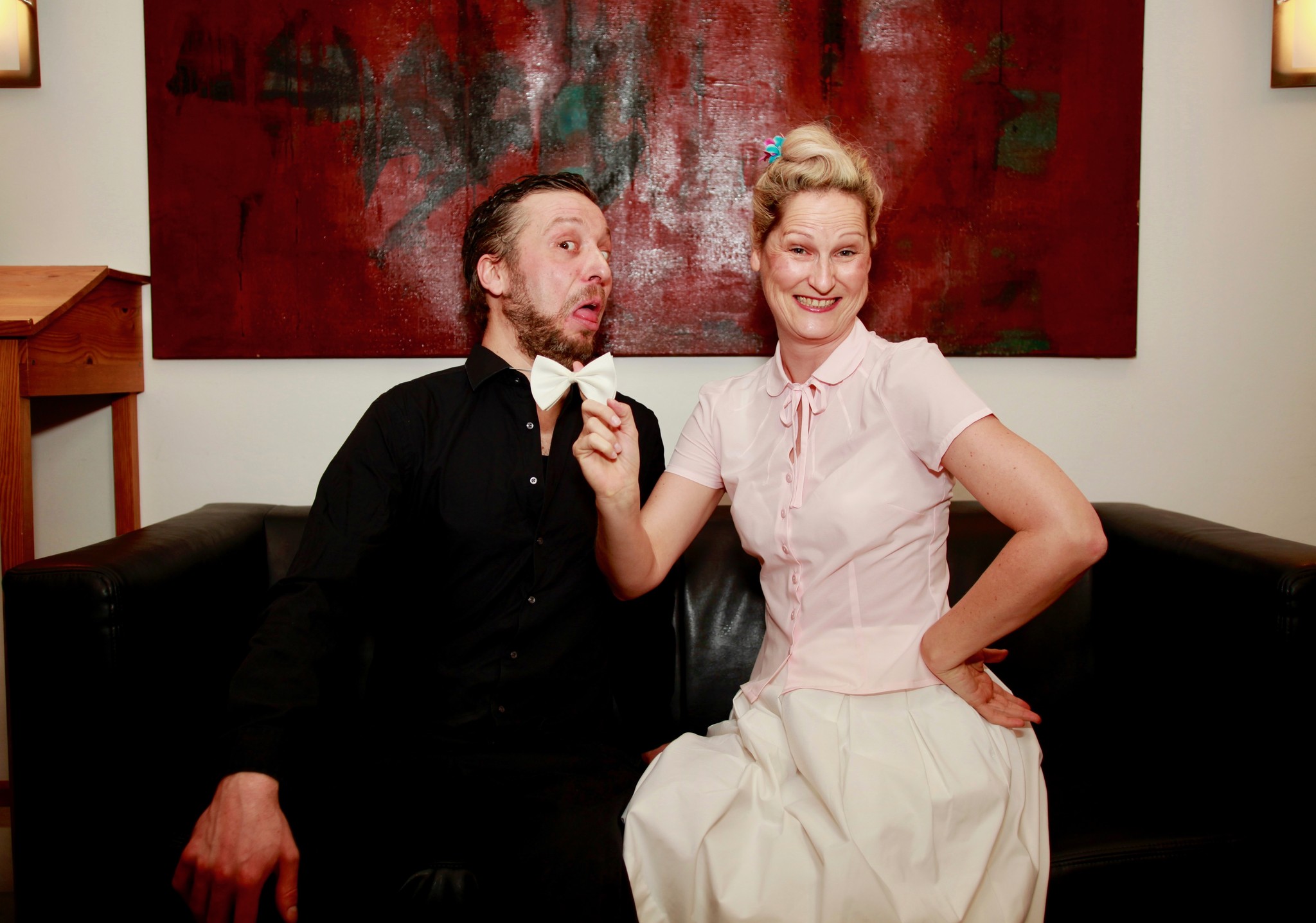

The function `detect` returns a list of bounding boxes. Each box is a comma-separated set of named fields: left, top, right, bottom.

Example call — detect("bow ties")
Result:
left=531, top=349, right=621, bottom=414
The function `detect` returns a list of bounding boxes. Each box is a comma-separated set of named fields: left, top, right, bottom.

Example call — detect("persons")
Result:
left=164, top=170, right=681, bottom=923
left=574, top=124, right=1108, bottom=923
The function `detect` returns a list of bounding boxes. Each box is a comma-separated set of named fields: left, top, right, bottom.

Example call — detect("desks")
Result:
left=0, top=264, right=155, bottom=571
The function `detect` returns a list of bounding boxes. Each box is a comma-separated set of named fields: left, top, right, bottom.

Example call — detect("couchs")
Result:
left=1, top=497, right=1316, bottom=923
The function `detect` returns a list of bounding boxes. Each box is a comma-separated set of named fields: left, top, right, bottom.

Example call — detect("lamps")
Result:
left=0, top=0, right=42, bottom=91
left=1265, top=0, right=1316, bottom=89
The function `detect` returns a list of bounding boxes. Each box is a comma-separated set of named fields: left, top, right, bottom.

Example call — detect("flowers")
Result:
left=758, top=132, right=788, bottom=166
